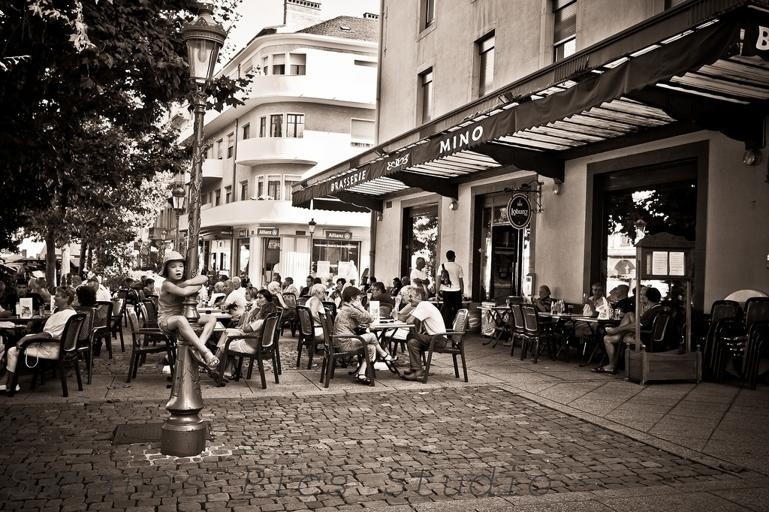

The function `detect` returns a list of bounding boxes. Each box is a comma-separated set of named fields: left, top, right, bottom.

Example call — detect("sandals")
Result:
left=188, top=346, right=206, bottom=366
left=203, top=351, right=220, bottom=367
left=353, top=373, right=370, bottom=384
left=379, top=353, right=398, bottom=362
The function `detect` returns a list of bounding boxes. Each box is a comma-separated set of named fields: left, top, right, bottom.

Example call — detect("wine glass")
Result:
left=582, top=292, right=589, bottom=300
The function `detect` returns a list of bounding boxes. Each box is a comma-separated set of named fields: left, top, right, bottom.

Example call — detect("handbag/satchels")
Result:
left=441, top=270, right=449, bottom=284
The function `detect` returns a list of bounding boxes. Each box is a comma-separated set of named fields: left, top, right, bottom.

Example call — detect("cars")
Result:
left=0, top=253, right=87, bottom=300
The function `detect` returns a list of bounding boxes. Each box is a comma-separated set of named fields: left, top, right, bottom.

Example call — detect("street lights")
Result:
left=154, top=7, right=232, bottom=458
left=170, top=184, right=186, bottom=249
left=306, top=216, right=317, bottom=275
left=161, top=230, right=167, bottom=243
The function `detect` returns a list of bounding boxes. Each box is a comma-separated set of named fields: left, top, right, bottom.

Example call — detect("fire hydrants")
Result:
left=137, top=237, right=143, bottom=268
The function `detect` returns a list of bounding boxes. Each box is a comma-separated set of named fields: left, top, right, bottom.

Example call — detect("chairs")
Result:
left=0, top=284, right=768, bottom=399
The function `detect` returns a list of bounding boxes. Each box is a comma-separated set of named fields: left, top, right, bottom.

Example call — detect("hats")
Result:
left=159, top=251, right=185, bottom=277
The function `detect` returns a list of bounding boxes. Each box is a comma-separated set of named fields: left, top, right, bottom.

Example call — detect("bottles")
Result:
left=551, top=298, right=565, bottom=315
left=38, top=305, right=45, bottom=316
left=200, top=298, right=229, bottom=315
left=15, top=301, right=23, bottom=316
left=610, top=300, right=621, bottom=321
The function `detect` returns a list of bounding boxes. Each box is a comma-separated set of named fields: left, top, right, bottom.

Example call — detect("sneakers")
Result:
left=404, top=368, right=425, bottom=381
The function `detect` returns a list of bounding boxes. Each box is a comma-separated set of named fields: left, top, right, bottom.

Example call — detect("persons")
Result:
left=434, top=251, right=463, bottom=340
left=556, top=283, right=664, bottom=374
left=504, top=285, right=552, bottom=346
left=134, top=250, right=277, bottom=381
left=268, top=257, right=448, bottom=385
left=1, top=258, right=132, bottom=390
